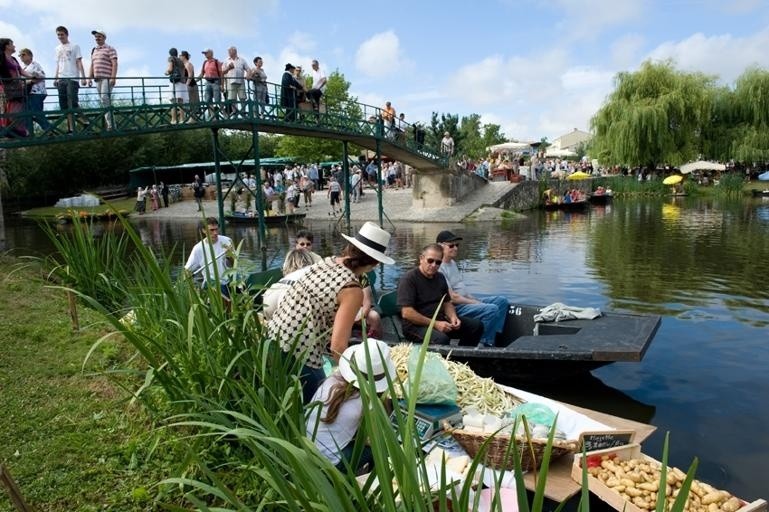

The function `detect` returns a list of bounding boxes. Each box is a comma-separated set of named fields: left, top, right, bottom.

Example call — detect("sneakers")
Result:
left=185, top=116, right=198, bottom=125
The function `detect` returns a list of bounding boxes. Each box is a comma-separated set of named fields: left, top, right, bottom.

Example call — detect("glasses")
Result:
left=300, top=243, right=310, bottom=246
left=443, top=242, right=459, bottom=248
left=18, top=54, right=25, bottom=57
left=428, top=259, right=441, bottom=265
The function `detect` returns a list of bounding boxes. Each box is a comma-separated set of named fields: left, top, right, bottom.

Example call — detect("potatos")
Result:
left=587, top=454, right=742, bottom=512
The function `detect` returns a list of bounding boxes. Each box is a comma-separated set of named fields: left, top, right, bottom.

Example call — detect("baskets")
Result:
left=442, top=414, right=581, bottom=471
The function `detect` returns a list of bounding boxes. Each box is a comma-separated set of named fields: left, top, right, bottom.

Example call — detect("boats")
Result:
left=57, top=210, right=129, bottom=224
left=193, top=286, right=661, bottom=378
left=225, top=214, right=305, bottom=224
left=752, top=189, right=769, bottom=196
left=542, top=192, right=615, bottom=213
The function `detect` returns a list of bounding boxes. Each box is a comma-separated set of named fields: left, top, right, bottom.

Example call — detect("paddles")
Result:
left=119, top=246, right=232, bottom=327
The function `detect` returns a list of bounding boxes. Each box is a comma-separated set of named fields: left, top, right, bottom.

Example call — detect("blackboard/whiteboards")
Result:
left=577, top=431, right=636, bottom=452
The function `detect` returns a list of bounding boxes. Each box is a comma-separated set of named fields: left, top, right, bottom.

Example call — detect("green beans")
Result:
left=391, top=341, right=520, bottom=416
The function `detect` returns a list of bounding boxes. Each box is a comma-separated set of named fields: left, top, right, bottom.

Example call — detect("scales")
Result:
left=387, top=399, right=462, bottom=441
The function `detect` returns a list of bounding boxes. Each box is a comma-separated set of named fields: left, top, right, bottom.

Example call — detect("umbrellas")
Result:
left=565, top=171, right=591, bottom=182
left=663, top=175, right=683, bottom=190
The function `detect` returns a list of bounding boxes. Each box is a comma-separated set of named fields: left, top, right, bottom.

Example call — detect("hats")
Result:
left=91, top=30, right=107, bottom=39
left=285, top=64, right=295, bottom=71
left=339, top=337, right=396, bottom=393
left=201, top=48, right=213, bottom=53
left=437, top=231, right=463, bottom=242
left=341, top=221, right=396, bottom=265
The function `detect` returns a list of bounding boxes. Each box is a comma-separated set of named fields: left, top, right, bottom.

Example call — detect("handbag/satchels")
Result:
left=170, top=57, right=181, bottom=83
left=4, top=80, right=24, bottom=101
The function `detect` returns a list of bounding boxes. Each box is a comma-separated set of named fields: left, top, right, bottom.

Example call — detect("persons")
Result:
left=180, top=217, right=235, bottom=300
left=0, top=25, right=118, bottom=138
left=165, top=47, right=326, bottom=124
left=460, top=153, right=565, bottom=182
left=543, top=187, right=613, bottom=205
left=368, top=102, right=455, bottom=159
left=565, top=159, right=593, bottom=175
left=262, top=221, right=511, bottom=476
left=135, top=154, right=412, bottom=214
